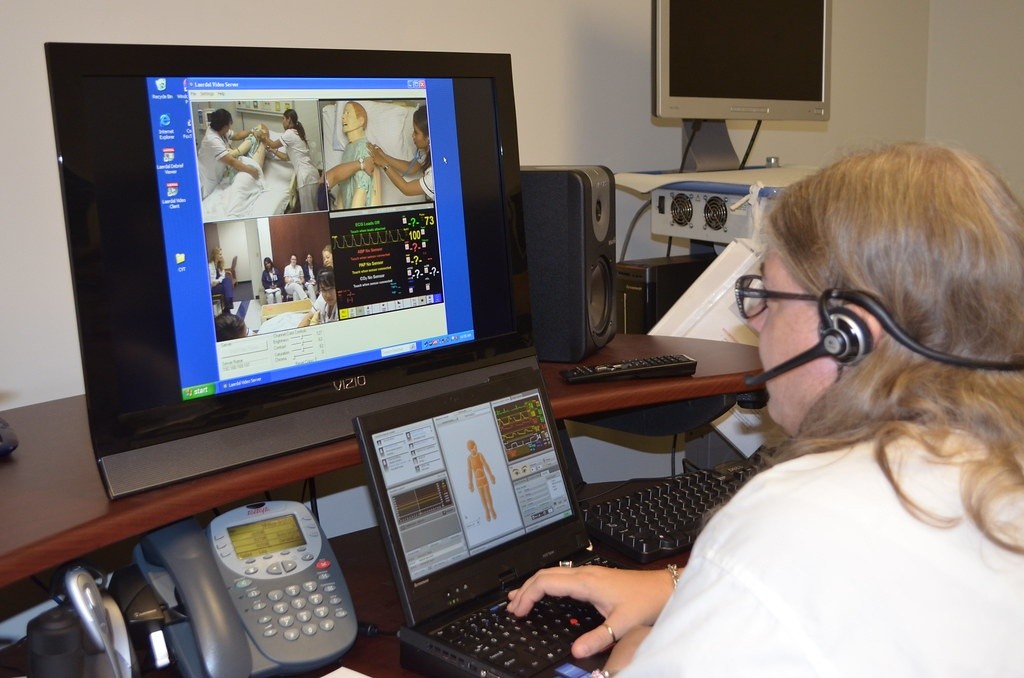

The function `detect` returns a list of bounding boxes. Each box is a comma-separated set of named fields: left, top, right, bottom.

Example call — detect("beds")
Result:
left=202, top=127, right=316, bottom=223
left=322, top=103, right=427, bottom=209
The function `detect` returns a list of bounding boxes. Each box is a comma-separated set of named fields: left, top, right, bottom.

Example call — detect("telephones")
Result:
left=135, top=498, right=363, bottom=677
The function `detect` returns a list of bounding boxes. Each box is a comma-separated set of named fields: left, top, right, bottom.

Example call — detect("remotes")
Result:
left=558, top=354, right=698, bottom=383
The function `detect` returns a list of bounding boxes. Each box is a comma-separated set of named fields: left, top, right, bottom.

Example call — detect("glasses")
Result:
left=735, top=275, right=820, bottom=319
left=318, top=286, right=336, bottom=293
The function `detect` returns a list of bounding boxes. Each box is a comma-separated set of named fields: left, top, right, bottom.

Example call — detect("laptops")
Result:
left=351, top=368, right=626, bottom=678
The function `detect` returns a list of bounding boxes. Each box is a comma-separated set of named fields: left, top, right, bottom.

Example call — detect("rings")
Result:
left=604, top=623, right=617, bottom=642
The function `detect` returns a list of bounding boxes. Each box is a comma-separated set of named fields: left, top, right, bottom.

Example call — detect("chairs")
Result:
left=224, top=255, right=238, bottom=288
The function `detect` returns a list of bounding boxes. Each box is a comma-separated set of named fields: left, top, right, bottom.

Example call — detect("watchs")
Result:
left=359, top=157, right=364, bottom=170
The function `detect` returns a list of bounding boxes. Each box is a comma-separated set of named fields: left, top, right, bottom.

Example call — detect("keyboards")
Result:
left=580, top=457, right=761, bottom=563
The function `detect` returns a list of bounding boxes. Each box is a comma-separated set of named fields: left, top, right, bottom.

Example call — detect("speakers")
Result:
left=523, top=164, right=618, bottom=366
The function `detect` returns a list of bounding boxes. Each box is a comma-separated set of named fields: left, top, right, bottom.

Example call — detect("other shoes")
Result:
left=226, top=301, right=233, bottom=310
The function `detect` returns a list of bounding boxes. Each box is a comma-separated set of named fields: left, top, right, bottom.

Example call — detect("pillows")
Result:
left=332, top=101, right=420, bottom=161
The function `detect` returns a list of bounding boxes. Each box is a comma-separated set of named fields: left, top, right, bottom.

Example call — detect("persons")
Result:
left=366, top=105, right=436, bottom=202
left=196, top=108, right=260, bottom=199
left=325, top=156, right=376, bottom=190
left=215, top=310, right=251, bottom=343
left=262, top=245, right=340, bottom=326
left=229, top=120, right=269, bottom=212
left=334, top=101, right=376, bottom=208
left=207, top=247, right=235, bottom=313
left=253, top=109, right=321, bottom=213
left=507, top=139, right=1020, bottom=678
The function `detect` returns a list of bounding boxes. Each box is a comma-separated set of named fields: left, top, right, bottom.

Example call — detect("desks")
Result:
left=0, top=336, right=765, bottom=678
left=261, top=298, right=314, bottom=319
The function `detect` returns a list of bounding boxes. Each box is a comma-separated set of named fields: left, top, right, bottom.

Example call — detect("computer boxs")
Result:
left=616, top=253, right=718, bottom=334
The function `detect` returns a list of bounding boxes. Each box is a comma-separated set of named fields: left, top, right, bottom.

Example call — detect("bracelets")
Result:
left=384, top=165, right=390, bottom=171
left=667, top=563, right=680, bottom=589
left=591, top=669, right=610, bottom=678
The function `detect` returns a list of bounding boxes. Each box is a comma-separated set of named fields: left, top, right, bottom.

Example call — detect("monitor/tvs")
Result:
left=44, top=42, right=544, bottom=499
left=650, top=0, right=833, bottom=171
